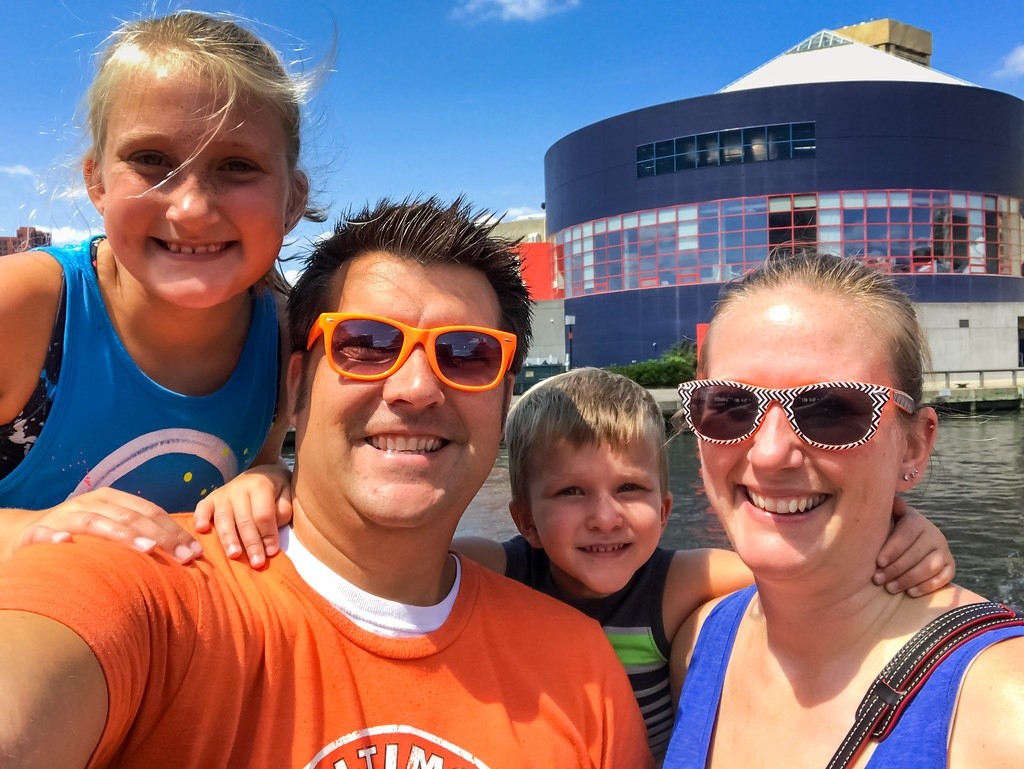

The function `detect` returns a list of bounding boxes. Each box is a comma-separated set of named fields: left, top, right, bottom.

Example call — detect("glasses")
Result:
left=677, top=379, right=915, bottom=452
left=307, top=312, right=517, bottom=392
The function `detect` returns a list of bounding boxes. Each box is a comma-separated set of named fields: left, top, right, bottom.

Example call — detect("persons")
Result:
left=661, top=246, right=1023, bottom=769
left=0, top=191, right=650, bottom=769
left=192, top=366, right=958, bottom=769
left=0, top=0, right=347, bottom=565
